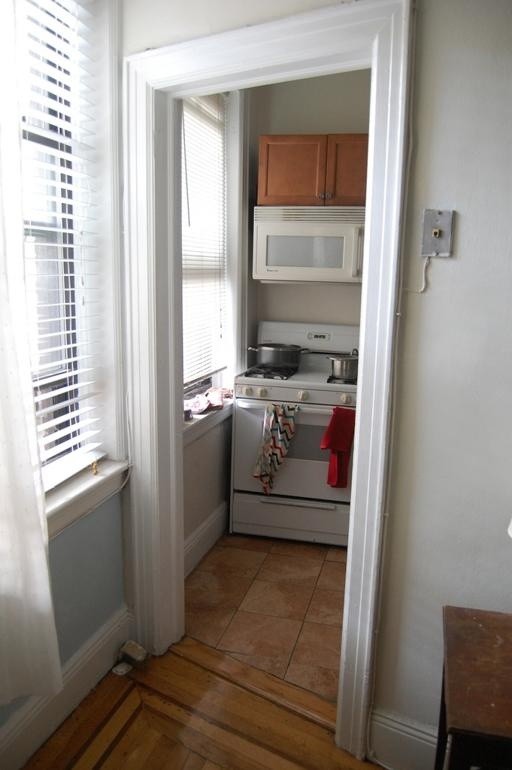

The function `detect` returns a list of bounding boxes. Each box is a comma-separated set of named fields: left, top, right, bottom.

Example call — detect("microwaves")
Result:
left=249, top=207, right=367, bottom=288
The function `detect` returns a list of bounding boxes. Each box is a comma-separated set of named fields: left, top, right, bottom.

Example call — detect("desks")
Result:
left=436, top=604, right=511, bottom=768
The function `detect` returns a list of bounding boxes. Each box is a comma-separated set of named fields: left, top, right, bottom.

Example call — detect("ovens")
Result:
left=229, top=399, right=359, bottom=550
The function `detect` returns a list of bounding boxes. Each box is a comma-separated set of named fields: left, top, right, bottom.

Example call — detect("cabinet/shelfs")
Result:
left=256, top=132, right=370, bottom=204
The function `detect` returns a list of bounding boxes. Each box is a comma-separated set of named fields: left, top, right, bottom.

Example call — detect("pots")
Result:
left=247, top=343, right=312, bottom=369
left=326, top=348, right=358, bottom=379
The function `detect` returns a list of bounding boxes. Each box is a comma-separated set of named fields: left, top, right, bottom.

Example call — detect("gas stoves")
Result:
left=233, top=367, right=357, bottom=407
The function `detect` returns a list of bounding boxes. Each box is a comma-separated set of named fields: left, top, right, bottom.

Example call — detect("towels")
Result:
left=250, top=404, right=355, bottom=497
